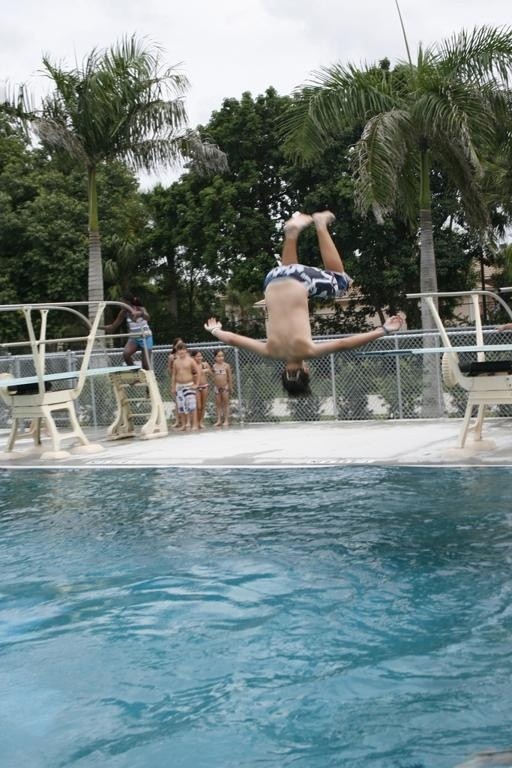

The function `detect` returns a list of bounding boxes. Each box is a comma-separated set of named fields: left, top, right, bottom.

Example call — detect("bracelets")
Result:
left=381, top=326, right=389, bottom=334
left=140, top=311, right=144, bottom=317
left=211, top=327, right=219, bottom=335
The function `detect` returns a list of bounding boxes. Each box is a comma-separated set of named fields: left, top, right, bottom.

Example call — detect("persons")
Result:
left=203, top=210, right=404, bottom=397
left=166, top=337, right=232, bottom=432
left=496, top=323, right=512, bottom=332
left=98, top=295, right=153, bottom=398
